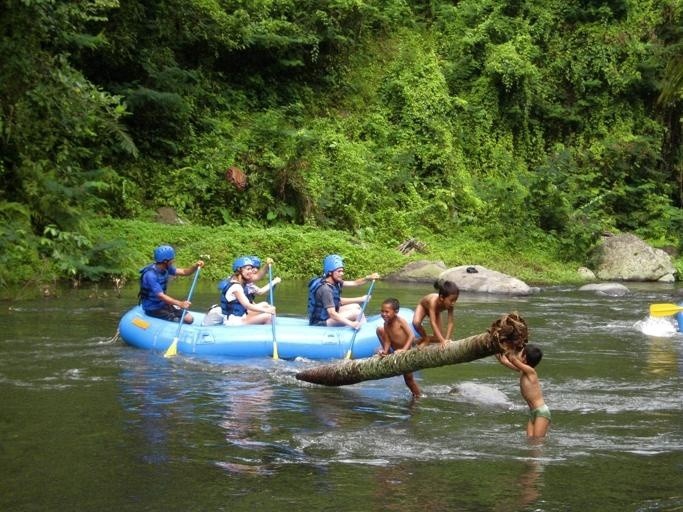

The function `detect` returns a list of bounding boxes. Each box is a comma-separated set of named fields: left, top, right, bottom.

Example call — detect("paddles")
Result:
left=166, top=265, right=200, bottom=355
left=269, top=263, right=278, bottom=360
left=650, top=304, right=683, bottom=316
left=345, top=279, right=376, bottom=360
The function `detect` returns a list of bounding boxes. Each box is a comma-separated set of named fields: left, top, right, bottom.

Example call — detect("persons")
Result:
left=320, top=254, right=381, bottom=306
left=306, top=259, right=372, bottom=332
left=241, top=254, right=282, bottom=305
left=216, top=255, right=277, bottom=328
left=410, top=278, right=461, bottom=352
left=374, top=297, right=421, bottom=399
left=495, top=342, right=552, bottom=438
left=136, top=243, right=204, bottom=326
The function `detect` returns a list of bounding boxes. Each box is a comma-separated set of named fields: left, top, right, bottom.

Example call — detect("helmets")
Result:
left=154, top=246, right=175, bottom=263
left=324, top=258, right=344, bottom=272
left=249, top=256, right=261, bottom=269
left=323, top=254, right=342, bottom=265
left=232, top=257, right=253, bottom=272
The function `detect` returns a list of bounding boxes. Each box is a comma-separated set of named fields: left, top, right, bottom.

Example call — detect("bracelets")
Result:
left=364, top=274, right=370, bottom=281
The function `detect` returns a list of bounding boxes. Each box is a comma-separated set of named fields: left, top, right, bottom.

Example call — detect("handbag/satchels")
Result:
left=200, top=304, right=223, bottom=325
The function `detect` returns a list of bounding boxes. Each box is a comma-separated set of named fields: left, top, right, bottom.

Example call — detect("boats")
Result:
left=119, top=304, right=421, bottom=363
